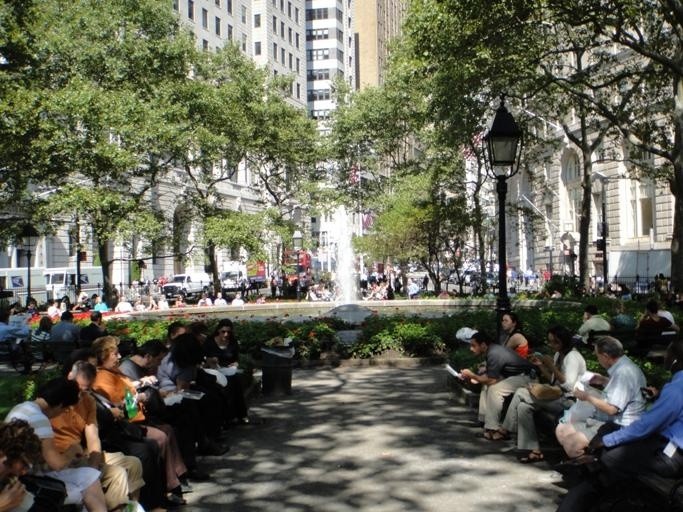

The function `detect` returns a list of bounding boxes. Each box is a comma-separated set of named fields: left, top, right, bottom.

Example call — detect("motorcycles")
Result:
left=511, top=270, right=537, bottom=288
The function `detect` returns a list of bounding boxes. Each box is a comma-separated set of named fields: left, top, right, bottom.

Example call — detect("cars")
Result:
left=430, top=266, right=497, bottom=296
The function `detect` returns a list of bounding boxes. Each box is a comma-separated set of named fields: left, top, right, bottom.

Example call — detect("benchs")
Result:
left=577, top=329, right=676, bottom=365
left=542, top=370, right=683, bottom=511
left=0, top=340, right=137, bottom=373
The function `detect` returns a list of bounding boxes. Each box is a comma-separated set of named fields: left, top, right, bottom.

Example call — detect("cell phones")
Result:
left=530, top=367, right=538, bottom=378
left=534, top=351, right=543, bottom=358
left=642, top=389, right=654, bottom=398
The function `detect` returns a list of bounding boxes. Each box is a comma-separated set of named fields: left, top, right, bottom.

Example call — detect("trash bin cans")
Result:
left=258, top=343, right=294, bottom=397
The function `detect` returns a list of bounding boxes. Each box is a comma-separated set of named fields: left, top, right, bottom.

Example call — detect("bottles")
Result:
left=124, top=387, right=138, bottom=420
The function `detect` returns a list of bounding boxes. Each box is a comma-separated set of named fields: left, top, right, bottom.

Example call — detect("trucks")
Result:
left=0, top=267, right=102, bottom=310
left=218, top=265, right=264, bottom=290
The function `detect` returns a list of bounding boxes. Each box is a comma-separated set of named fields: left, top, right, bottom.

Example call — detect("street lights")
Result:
left=292, top=230, right=302, bottom=302
left=484, top=95, right=523, bottom=343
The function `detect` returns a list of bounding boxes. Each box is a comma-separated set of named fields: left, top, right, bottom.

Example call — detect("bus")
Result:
left=280, top=249, right=311, bottom=290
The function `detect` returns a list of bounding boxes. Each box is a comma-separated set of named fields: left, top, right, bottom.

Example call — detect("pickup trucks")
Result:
left=165, top=274, right=213, bottom=298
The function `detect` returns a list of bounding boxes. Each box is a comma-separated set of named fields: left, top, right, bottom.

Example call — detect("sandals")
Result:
left=520, top=450, right=546, bottom=464
left=483, top=428, right=512, bottom=442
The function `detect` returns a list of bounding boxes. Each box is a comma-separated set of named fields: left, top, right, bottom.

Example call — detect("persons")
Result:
left=510, top=267, right=549, bottom=288
left=198, top=291, right=265, bottom=306
left=271, top=270, right=335, bottom=301
left=361, top=275, right=461, bottom=300
left=537, top=284, right=631, bottom=300
left=2, top=318, right=251, bottom=511
left=462, top=302, right=683, bottom=512
left=131, top=278, right=186, bottom=311
left=1, top=289, right=131, bottom=370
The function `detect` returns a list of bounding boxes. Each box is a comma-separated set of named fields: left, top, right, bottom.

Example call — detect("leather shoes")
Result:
left=554, top=456, right=596, bottom=473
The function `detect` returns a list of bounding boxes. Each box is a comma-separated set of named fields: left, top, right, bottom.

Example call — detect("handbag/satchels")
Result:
left=117, top=419, right=143, bottom=441
left=20, top=472, right=67, bottom=512
left=136, top=383, right=170, bottom=426
left=530, top=382, right=564, bottom=402
